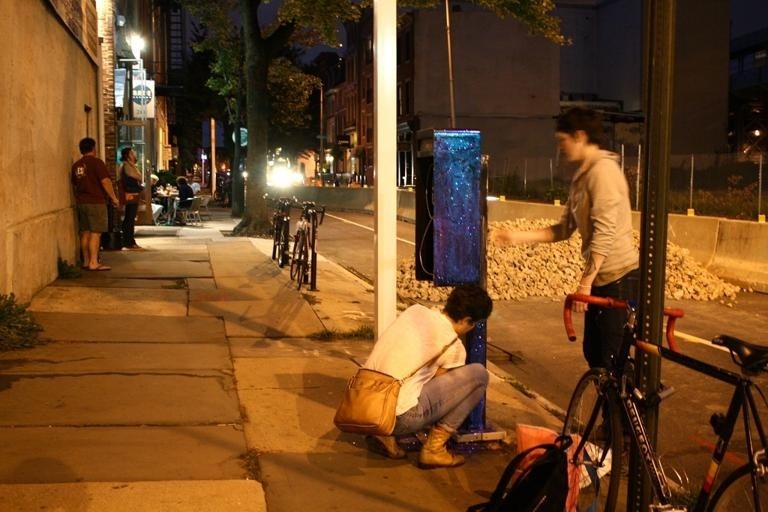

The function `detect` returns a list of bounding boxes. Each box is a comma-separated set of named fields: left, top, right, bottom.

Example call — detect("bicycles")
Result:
left=261, top=192, right=326, bottom=291
left=498, top=293, right=768, bottom=511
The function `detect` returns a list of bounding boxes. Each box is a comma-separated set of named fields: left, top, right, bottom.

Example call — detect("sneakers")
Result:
left=127, top=242, right=142, bottom=248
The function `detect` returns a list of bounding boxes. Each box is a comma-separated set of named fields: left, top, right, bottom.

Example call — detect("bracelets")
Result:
left=578, top=282, right=592, bottom=289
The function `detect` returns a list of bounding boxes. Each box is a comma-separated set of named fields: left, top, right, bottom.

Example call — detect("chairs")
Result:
left=200, top=195, right=211, bottom=208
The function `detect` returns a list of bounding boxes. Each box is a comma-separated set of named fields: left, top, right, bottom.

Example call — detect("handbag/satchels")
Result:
left=333, top=368, right=403, bottom=436
left=120, top=164, right=144, bottom=193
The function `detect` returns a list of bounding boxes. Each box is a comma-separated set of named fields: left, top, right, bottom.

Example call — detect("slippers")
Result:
left=81, top=263, right=111, bottom=270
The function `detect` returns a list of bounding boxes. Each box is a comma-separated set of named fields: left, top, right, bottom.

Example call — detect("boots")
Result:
left=418, top=423, right=465, bottom=469
left=366, top=435, right=407, bottom=459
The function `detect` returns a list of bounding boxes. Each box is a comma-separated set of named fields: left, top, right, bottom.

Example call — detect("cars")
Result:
left=204, top=170, right=231, bottom=189
left=239, top=160, right=247, bottom=184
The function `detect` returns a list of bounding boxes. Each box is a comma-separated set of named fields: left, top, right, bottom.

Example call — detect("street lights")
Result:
left=288, top=75, right=324, bottom=173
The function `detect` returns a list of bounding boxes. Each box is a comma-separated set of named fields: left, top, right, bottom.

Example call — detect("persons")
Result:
left=492, top=112, right=639, bottom=445
left=72, top=138, right=120, bottom=271
left=365, top=285, right=493, bottom=469
left=151, top=174, right=193, bottom=225
left=120, top=147, right=143, bottom=250
